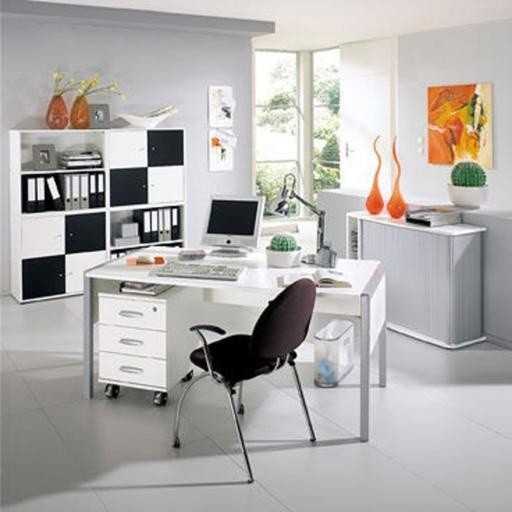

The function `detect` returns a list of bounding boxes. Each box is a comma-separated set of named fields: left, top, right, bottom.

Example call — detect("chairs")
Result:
left=175, top=279, right=316, bottom=482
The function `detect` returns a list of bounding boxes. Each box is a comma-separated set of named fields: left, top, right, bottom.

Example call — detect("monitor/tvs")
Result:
left=201, top=195, right=267, bottom=257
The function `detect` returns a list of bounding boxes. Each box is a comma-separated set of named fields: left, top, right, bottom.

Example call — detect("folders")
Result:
left=22, top=172, right=105, bottom=213
left=133, top=207, right=180, bottom=243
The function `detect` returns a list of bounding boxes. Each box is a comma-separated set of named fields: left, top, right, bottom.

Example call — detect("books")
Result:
left=59, top=148, right=103, bottom=170
left=118, top=284, right=174, bottom=296
left=276, top=269, right=352, bottom=287
left=123, top=280, right=155, bottom=289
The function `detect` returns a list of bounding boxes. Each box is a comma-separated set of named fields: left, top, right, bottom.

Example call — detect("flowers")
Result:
left=53, top=71, right=128, bottom=101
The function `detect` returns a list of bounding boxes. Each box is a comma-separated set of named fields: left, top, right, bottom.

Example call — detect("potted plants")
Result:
left=266, top=233, right=302, bottom=268
left=447, top=161, right=489, bottom=208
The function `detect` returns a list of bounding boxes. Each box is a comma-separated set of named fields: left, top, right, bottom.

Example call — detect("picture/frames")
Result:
left=87, top=104, right=111, bottom=129
left=207, top=85, right=236, bottom=172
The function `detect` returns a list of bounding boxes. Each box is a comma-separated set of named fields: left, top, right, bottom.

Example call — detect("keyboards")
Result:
left=154, top=261, right=248, bottom=282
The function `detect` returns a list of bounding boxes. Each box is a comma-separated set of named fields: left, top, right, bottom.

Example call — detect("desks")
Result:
left=84, top=245, right=387, bottom=443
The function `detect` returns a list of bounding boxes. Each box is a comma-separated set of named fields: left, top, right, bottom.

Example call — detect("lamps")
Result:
left=265, top=173, right=337, bottom=268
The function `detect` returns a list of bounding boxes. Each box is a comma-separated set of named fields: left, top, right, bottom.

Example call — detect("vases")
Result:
left=46, top=96, right=90, bottom=129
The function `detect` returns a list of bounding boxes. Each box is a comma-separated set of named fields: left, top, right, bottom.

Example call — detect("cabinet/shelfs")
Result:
left=8, top=129, right=185, bottom=306
left=96, top=289, right=195, bottom=394
left=345, top=209, right=487, bottom=351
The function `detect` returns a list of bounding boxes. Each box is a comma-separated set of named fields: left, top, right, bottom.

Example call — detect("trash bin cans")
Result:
left=314, top=319, right=355, bottom=388
